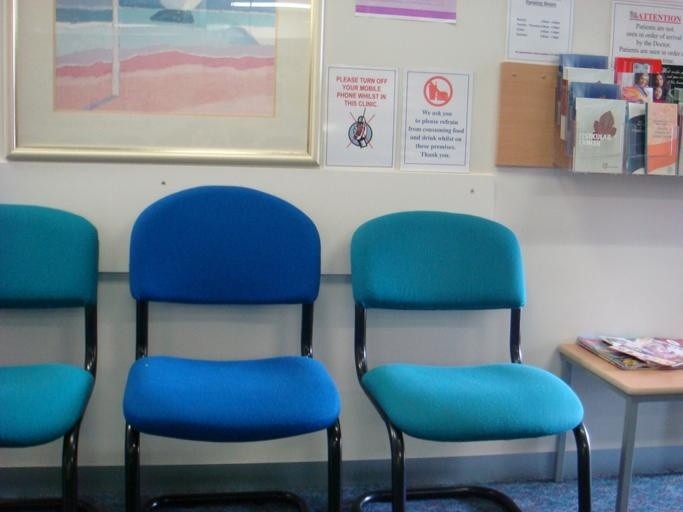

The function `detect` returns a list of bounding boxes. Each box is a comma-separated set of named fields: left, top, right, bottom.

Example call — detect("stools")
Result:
left=555, top=341, right=683, bottom=511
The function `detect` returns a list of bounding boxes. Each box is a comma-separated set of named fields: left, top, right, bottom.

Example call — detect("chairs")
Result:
left=2, top=204, right=100, bottom=508
left=349, top=210, right=591, bottom=512
left=122, top=183, right=344, bottom=511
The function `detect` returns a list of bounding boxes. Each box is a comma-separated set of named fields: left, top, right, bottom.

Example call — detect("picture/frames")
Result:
left=0, top=0, right=325, bottom=168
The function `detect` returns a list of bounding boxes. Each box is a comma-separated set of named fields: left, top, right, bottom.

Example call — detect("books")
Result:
left=574, top=333, right=683, bottom=372
left=551, top=51, right=682, bottom=177
